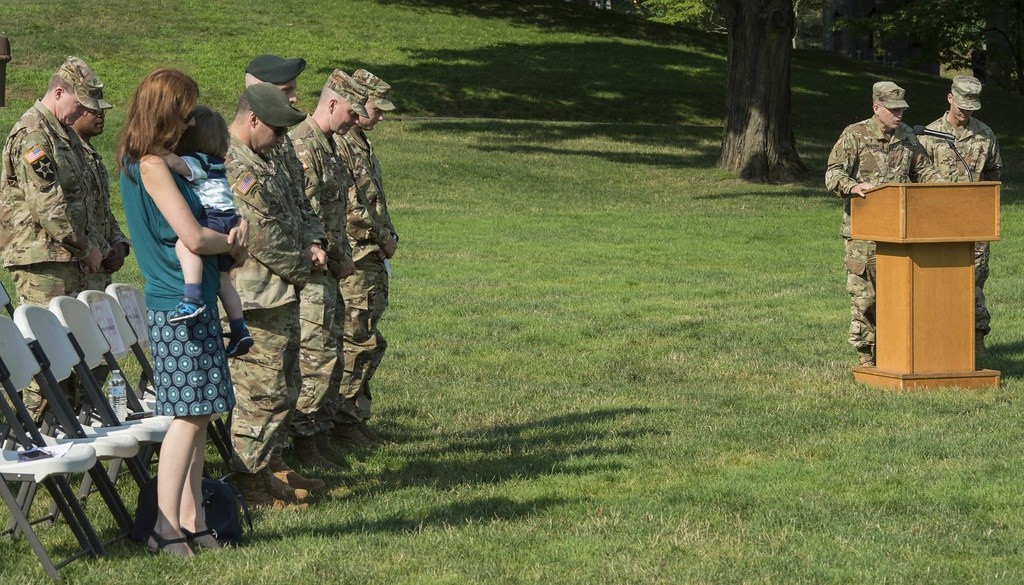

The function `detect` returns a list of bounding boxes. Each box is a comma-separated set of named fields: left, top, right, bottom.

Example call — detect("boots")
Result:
left=241, top=480, right=308, bottom=512
left=316, top=432, right=346, bottom=465
left=265, top=475, right=312, bottom=503
left=857, top=347, right=875, bottom=367
left=335, top=421, right=384, bottom=450
left=294, top=437, right=341, bottom=471
left=270, top=457, right=322, bottom=489
left=975, top=330, right=988, bottom=357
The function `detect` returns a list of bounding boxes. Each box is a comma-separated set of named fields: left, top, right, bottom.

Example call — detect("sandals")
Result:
left=145, top=530, right=187, bottom=558
left=183, top=528, right=213, bottom=553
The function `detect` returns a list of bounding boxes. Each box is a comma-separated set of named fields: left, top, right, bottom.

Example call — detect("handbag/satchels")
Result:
left=131, top=474, right=242, bottom=547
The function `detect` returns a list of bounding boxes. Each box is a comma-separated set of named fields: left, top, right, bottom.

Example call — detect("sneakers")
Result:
left=225, top=329, right=254, bottom=357
left=169, top=297, right=206, bottom=322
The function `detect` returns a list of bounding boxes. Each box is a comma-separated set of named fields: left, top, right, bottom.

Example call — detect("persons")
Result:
left=119, top=70, right=249, bottom=560
left=285, top=67, right=368, bottom=464
left=826, top=79, right=947, bottom=368
left=215, top=52, right=330, bottom=511
left=0, top=56, right=133, bottom=447
left=153, top=104, right=256, bottom=360
left=908, top=71, right=1004, bottom=364
left=322, top=68, right=401, bottom=448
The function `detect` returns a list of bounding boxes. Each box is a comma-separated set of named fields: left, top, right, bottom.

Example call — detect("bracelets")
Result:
left=390, top=231, right=400, bottom=241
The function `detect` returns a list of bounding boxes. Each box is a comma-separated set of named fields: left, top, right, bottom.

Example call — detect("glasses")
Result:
left=182, top=108, right=197, bottom=124
left=273, top=126, right=289, bottom=136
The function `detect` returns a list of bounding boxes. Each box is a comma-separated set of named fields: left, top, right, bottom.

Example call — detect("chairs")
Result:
left=0, top=280, right=233, bottom=585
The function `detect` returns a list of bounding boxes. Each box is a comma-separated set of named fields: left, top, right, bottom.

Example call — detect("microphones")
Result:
left=913, top=125, right=956, bottom=140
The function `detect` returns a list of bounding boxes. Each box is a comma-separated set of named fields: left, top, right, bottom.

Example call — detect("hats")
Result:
left=873, top=81, right=909, bottom=109
left=324, top=69, right=369, bottom=118
left=247, top=82, right=307, bottom=127
left=59, top=55, right=104, bottom=110
left=951, top=75, right=982, bottom=111
left=352, top=69, right=396, bottom=111
left=245, top=54, right=306, bottom=84
left=97, top=95, right=113, bottom=109
